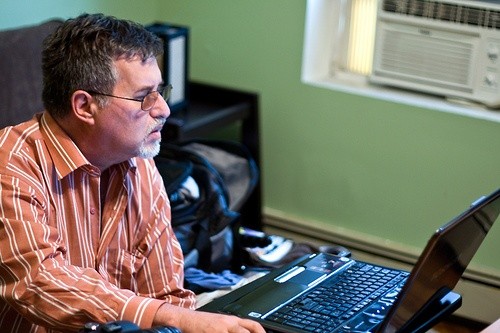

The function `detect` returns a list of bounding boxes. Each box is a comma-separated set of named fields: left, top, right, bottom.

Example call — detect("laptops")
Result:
left=222, top=189, right=497, bottom=333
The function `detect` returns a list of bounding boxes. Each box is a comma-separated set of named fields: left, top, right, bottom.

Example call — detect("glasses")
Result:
left=88, top=83, right=173, bottom=111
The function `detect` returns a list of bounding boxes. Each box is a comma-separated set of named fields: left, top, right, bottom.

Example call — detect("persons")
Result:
left=0, top=12, right=268, bottom=333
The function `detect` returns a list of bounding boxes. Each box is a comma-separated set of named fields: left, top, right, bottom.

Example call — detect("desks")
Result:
left=162, top=81, right=262, bottom=233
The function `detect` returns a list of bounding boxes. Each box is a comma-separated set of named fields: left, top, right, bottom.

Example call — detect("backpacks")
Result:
left=153, top=138, right=259, bottom=296
